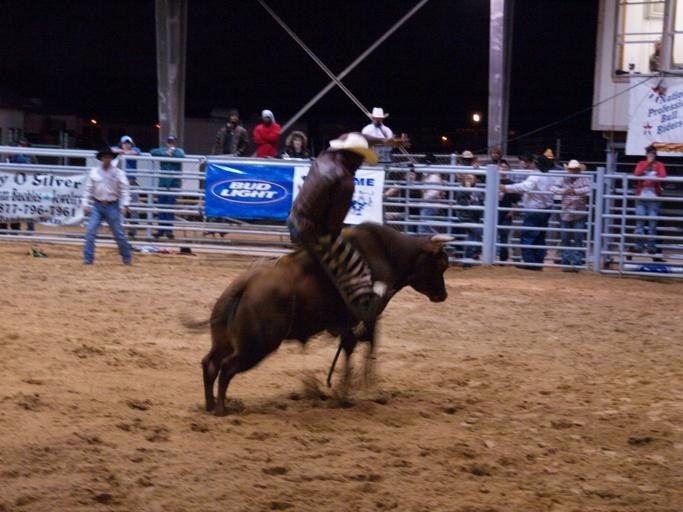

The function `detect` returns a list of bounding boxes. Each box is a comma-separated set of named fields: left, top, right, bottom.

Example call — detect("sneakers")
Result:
left=124, top=231, right=176, bottom=239
left=463, top=247, right=661, bottom=269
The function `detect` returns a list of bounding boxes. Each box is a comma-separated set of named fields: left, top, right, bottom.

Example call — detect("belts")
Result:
left=91, top=196, right=119, bottom=205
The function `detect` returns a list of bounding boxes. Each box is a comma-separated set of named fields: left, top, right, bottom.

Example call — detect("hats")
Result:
left=284, top=130, right=309, bottom=149
left=96, top=146, right=120, bottom=161
left=167, top=135, right=178, bottom=142
left=563, top=159, right=587, bottom=172
left=329, top=133, right=379, bottom=167
left=543, top=148, right=554, bottom=160
left=461, top=150, right=474, bottom=159
left=120, top=136, right=134, bottom=144
left=367, top=106, right=390, bottom=120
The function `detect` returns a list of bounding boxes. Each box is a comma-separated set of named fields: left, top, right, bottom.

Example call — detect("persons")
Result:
left=0, top=140, right=24, bottom=234
left=106, top=136, right=142, bottom=239
left=147, top=136, right=183, bottom=238
left=16, top=138, right=37, bottom=232
left=250, top=110, right=279, bottom=157
left=282, top=132, right=410, bottom=341
left=210, top=110, right=249, bottom=157
left=361, top=107, right=394, bottom=163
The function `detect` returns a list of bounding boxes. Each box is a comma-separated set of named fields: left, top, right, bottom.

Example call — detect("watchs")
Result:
left=81, top=144, right=130, bottom=265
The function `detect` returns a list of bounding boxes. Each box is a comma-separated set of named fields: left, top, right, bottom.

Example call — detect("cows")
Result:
left=177, top=220, right=455, bottom=418
